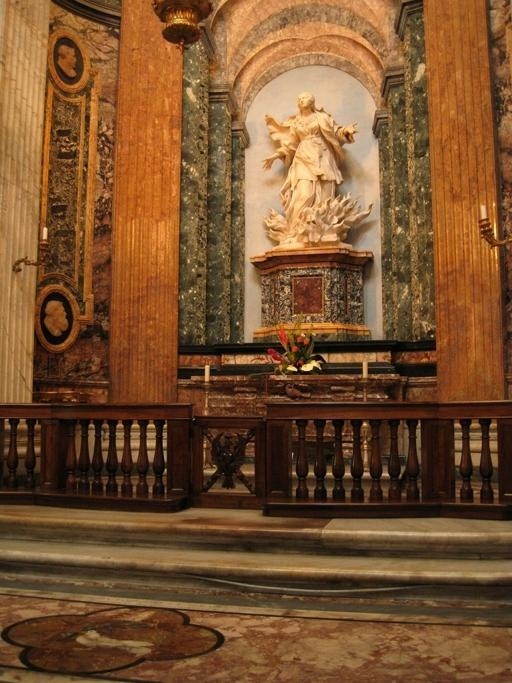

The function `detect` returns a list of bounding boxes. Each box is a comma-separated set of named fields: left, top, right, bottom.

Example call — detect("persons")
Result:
left=261, top=91, right=360, bottom=212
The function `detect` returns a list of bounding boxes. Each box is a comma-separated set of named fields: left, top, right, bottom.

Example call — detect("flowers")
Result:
left=250, top=310, right=326, bottom=375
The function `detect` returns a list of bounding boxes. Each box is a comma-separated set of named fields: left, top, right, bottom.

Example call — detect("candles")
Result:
left=42, top=225, right=49, bottom=240
left=362, top=359, right=369, bottom=378
left=204, top=364, right=210, bottom=383
left=479, top=204, right=487, bottom=220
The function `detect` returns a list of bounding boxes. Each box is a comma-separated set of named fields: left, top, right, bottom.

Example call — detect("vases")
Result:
left=285, top=383, right=313, bottom=400
